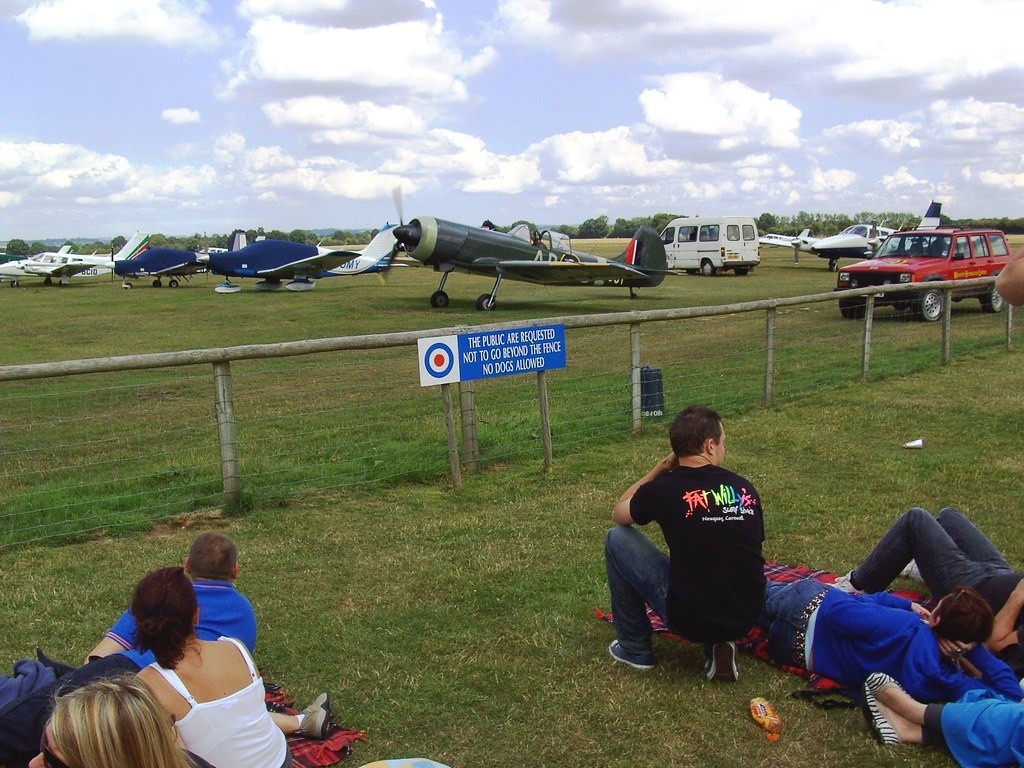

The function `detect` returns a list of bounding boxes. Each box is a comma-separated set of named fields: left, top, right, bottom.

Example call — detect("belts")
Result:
left=793, top=590, right=829, bottom=670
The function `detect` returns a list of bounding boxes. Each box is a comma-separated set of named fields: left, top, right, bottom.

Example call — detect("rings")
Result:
left=955, top=649, right=962, bottom=654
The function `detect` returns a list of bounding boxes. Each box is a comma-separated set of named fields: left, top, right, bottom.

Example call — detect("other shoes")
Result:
left=861, top=683, right=899, bottom=746
left=866, top=672, right=923, bottom=708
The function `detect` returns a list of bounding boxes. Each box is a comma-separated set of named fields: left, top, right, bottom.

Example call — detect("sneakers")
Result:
left=705, top=639, right=740, bottom=683
left=824, top=569, right=864, bottom=595
left=294, top=693, right=331, bottom=739
left=902, top=560, right=923, bottom=582
left=608, top=637, right=657, bottom=669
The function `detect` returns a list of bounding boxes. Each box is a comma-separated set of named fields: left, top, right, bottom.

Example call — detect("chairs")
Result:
left=709, top=229, right=715, bottom=236
left=910, top=243, right=923, bottom=254
left=701, top=231, right=707, bottom=235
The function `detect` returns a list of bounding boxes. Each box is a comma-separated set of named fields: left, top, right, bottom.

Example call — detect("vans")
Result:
left=660, top=216, right=761, bottom=277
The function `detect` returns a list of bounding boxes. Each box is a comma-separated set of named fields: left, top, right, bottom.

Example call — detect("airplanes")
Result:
left=0, top=245, right=114, bottom=287
left=810, top=224, right=897, bottom=272
left=16, top=232, right=152, bottom=287
left=863, top=202, right=981, bottom=260
left=758, top=228, right=820, bottom=251
left=103, top=230, right=247, bottom=290
left=383, top=184, right=668, bottom=311
left=199, top=224, right=419, bottom=294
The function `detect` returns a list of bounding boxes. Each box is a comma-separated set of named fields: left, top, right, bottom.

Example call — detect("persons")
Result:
left=0, top=531, right=257, bottom=767
left=762, top=576, right=1024, bottom=706
left=994, top=244, right=1024, bottom=307
left=858, top=671, right=1024, bottom=768
left=602, top=405, right=769, bottom=683
left=529, top=230, right=548, bottom=250
left=824, top=507, right=1024, bottom=679
left=129, top=567, right=333, bottom=768
left=27, top=673, right=189, bottom=768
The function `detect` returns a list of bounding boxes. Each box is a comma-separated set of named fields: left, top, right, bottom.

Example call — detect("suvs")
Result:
left=833, top=226, right=1013, bottom=322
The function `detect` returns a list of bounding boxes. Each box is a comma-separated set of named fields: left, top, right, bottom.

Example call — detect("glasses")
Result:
left=39, top=730, right=71, bottom=768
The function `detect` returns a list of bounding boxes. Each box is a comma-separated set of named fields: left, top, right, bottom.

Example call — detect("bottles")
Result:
left=902, top=439, right=923, bottom=449
left=750, top=697, right=783, bottom=733
